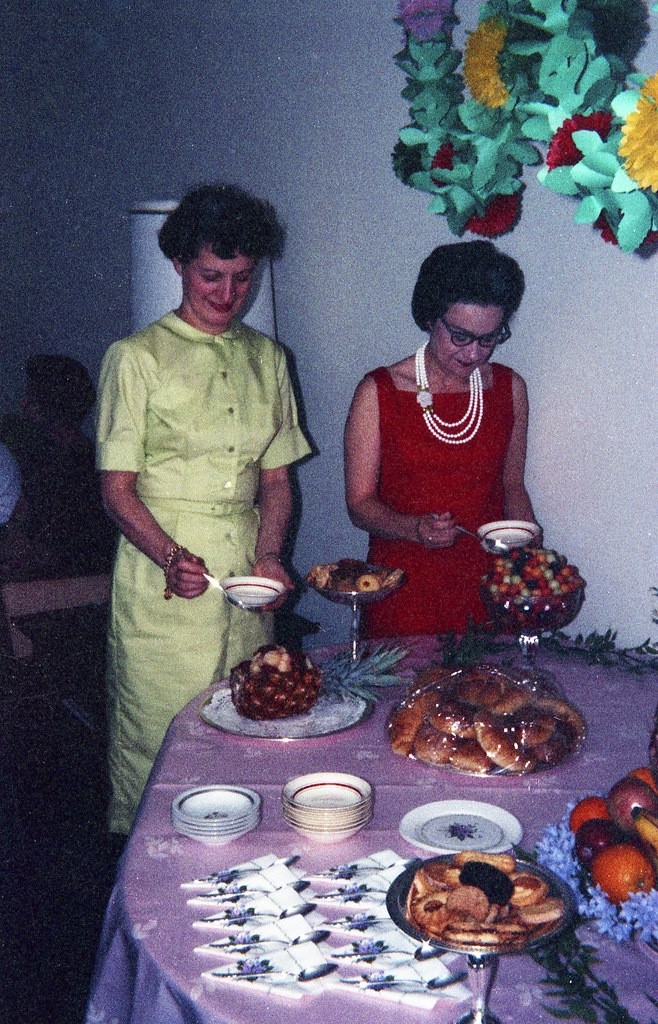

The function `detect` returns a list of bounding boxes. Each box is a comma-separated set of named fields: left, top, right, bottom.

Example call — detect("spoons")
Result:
left=211, top=964, right=339, bottom=983
left=197, top=880, right=311, bottom=903
left=208, top=930, right=331, bottom=947
left=199, top=903, right=317, bottom=923
left=204, top=572, right=244, bottom=608
left=363, top=967, right=469, bottom=992
left=339, top=939, right=450, bottom=983
left=431, top=512, right=509, bottom=556
left=193, top=855, right=302, bottom=887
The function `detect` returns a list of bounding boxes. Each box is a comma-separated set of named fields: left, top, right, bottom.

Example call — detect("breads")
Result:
left=385, top=663, right=585, bottom=775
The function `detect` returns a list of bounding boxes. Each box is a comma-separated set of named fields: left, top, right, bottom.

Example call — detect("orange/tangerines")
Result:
left=589, top=843, right=656, bottom=905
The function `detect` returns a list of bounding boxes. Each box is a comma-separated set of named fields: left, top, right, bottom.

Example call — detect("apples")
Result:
left=608, top=776, right=656, bottom=835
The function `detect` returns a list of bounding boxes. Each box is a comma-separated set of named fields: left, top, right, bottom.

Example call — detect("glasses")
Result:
left=436, top=312, right=512, bottom=347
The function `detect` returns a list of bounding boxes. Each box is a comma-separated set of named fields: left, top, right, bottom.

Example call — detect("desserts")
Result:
left=405, top=851, right=565, bottom=944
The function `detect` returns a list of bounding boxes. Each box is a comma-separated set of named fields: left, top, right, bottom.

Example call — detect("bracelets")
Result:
left=252, top=550, right=282, bottom=566
left=161, top=544, right=185, bottom=600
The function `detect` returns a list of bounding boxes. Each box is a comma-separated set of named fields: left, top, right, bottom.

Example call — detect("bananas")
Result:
left=631, top=807, right=658, bottom=857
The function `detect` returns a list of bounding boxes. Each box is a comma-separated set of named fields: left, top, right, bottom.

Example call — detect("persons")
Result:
left=1, top=353, right=115, bottom=699
left=101, top=184, right=311, bottom=860
left=342, top=239, right=543, bottom=643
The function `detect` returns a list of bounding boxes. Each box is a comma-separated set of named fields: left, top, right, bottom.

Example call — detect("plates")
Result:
left=199, top=687, right=374, bottom=740
left=398, top=799, right=524, bottom=856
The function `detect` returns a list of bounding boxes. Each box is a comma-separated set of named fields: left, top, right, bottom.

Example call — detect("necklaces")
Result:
left=415, top=339, right=485, bottom=444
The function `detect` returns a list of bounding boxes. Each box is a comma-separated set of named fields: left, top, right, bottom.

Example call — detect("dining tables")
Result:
left=88, top=637, right=657, bottom=1024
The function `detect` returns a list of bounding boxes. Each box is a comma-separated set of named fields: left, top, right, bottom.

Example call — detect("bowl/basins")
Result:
left=476, top=520, right=542, bottom=549
left=219, top=575, right=288, bottom=607
left=171, top=784, right=262, bottom=846
left=282, top=772, right=374, bottom=845
